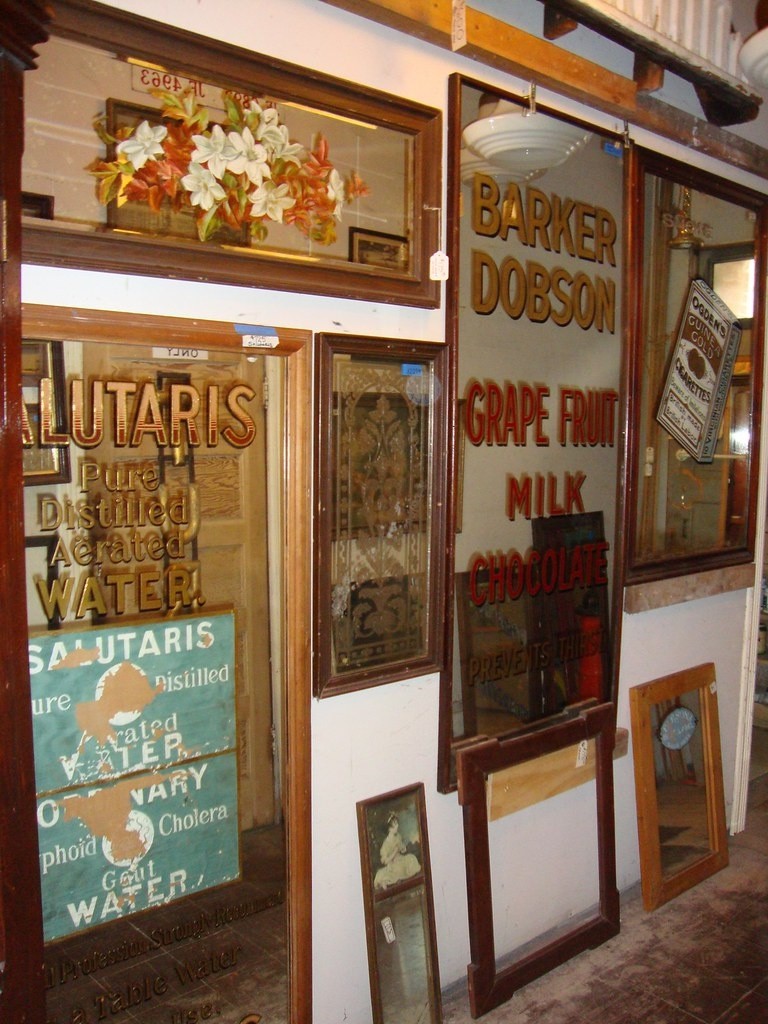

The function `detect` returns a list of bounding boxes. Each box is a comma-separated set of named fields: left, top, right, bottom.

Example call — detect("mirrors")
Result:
left=629, top=661, right=727, bottom=911
left=436, top=72, right=634, bottom=797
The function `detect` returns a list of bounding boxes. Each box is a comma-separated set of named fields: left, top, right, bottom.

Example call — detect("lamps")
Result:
left=460, top=92, right=593, bottom=187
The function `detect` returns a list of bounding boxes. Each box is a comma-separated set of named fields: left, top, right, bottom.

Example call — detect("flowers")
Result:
left=85, top=86, right=366, bottom=250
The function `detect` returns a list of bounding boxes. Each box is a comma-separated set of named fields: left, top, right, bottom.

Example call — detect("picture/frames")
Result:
left=22, top=339, right=72, bottom=488
left=24, top=0, right=441, bottom=312
left=626, top=142, right=768, bottom=591
left=358, top=780, right=443, bottom=1023
left=22, top=301, right=314, bottom=1024
left=458, top=702, right=621, bottom=1021
left=315, top=331, right=443, bottom=700
